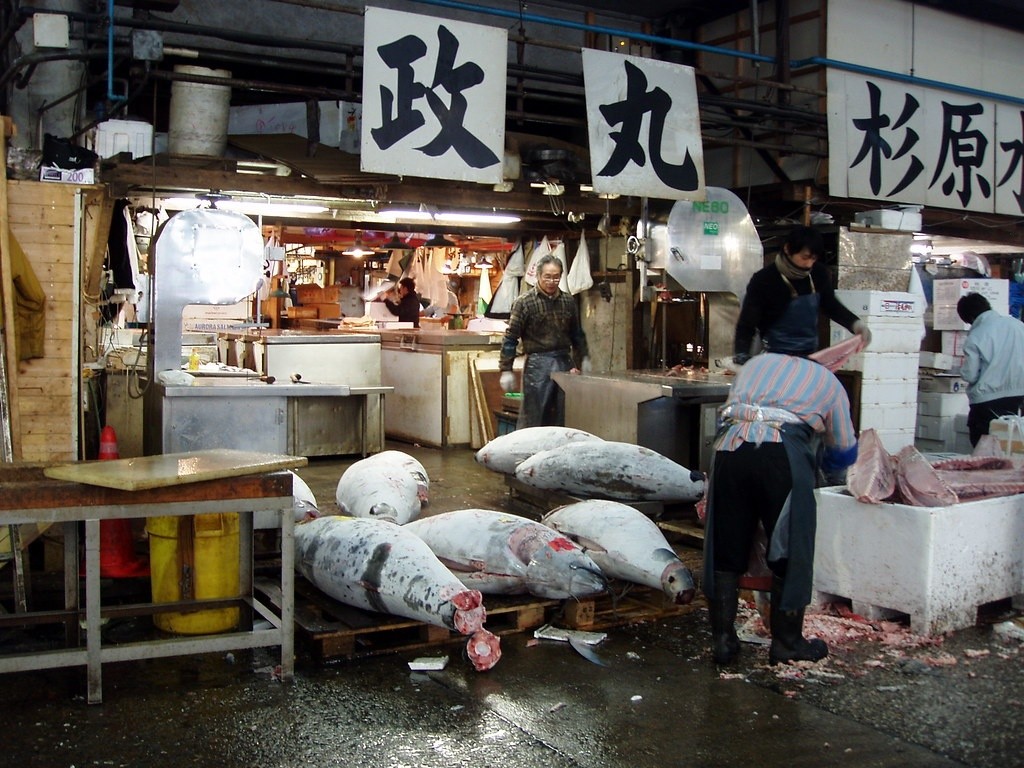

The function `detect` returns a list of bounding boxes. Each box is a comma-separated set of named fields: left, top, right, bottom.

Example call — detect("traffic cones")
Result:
left=79, top=426, right=152, bottom=577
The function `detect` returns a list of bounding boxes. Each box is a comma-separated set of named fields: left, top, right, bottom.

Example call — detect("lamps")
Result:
left=474, top=254, right=493, bottom=268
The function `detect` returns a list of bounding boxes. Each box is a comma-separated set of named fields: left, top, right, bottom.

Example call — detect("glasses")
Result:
left=540, top=274, right=560, bottom=285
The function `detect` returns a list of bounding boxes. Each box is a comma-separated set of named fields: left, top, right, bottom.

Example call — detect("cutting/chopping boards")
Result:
left=43, top=449, right=308, bottom=492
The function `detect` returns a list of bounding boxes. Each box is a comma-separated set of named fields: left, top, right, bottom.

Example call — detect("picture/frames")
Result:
left=464, top=265, right=471, bottom=274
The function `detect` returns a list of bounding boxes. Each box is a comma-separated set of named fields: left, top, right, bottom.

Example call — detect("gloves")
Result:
left=852, top=320, right=872, bottom=348
left=733, top=352, right=752, bottom=365
left=581, top=355, right=593, bottom=372
left=377, top=291, right=386, bottom=302
left=500, top=371, right=517, bottom=392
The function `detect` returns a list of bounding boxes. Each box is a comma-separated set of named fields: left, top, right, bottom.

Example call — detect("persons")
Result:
left=499, top=253, right=590, bottom=431
left=956, top=290, right=1024, bottom=450
left=734, top=225, right=872, bottom=369
left=418, top=285, right=463, bottom=322
left=377, top=277, right=419, bottom=328
left=706, top=351, right=861, bottom=666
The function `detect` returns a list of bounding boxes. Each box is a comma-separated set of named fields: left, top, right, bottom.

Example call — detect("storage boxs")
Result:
left=419, top=316, right=448, bottom=331
left=831, top=210, right=1024, bottom=468
left=288, top=283, right=342, bottom=319
left=81, top=118, right=153, bottom=160
left=811, top=485, right=1024, bottom=633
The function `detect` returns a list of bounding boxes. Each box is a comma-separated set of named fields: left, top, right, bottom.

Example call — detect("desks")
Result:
left=0, top=460, right=296, bottom=705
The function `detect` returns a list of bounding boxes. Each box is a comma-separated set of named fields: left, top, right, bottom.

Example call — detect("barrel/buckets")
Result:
left=146, top=512, right=240, bottom=635
left=167, top=62, right=232, bottom=158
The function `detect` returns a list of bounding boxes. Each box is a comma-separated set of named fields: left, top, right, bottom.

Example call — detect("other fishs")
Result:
left=283, top=335, right=1024, bottom=674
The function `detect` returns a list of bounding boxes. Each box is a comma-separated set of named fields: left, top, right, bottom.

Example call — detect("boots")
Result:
left=769, top=601, right=828, bottom=667
left=707, top=590, right=741, bottom=667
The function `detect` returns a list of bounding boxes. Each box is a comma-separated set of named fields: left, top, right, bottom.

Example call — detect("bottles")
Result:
left=188, top=349, right=200, bottom=371
